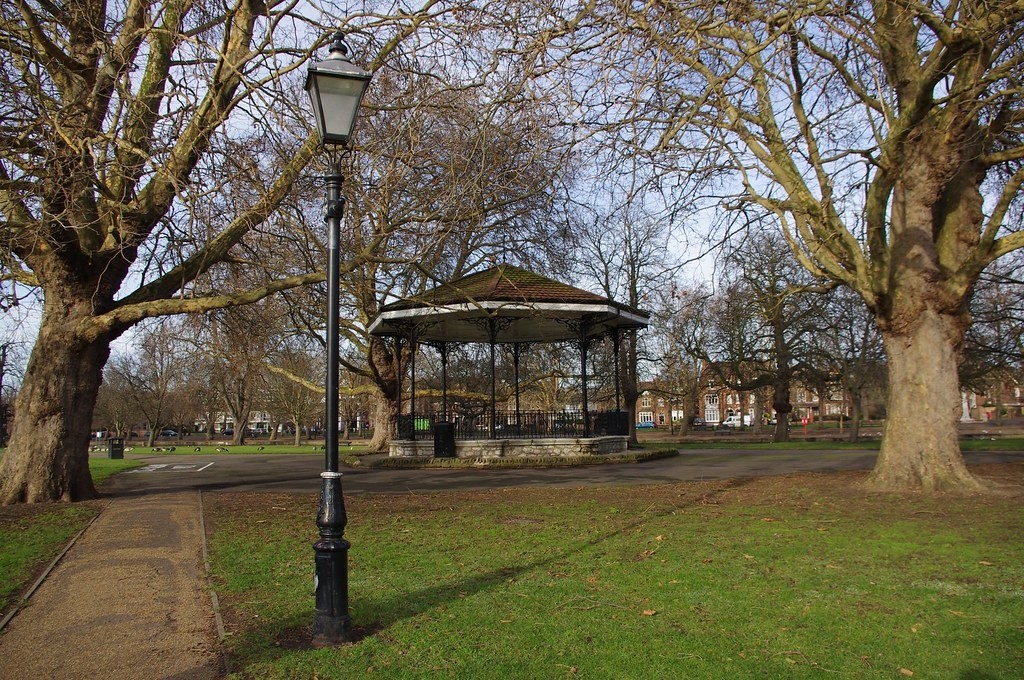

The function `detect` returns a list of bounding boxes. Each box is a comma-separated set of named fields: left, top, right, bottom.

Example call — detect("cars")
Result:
left=130, top=433, right=138, bottom=437
left=482, top=423, right=504, bottom=432
left=160, top=430, right=177, bottom=436
left=693, top=417, right=707, bottom=426
left=144, top=432, right=151, bottom=436
left=635, top=421, right=658, bottom=430
left=768, top=418, right=778, bottom=425
left=222, top=428, right=234, bottom=435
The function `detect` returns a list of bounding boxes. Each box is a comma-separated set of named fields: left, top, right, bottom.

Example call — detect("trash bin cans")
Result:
left=108, top=437, right=124, bottom=459
left=433, top=422, right=456, bottom=457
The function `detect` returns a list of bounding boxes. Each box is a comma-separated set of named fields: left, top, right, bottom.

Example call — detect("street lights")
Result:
left=303, top=29, right=379, bottom=644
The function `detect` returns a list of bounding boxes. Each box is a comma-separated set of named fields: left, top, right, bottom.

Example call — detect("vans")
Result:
left=722, top=415, right=753, bottom=428
